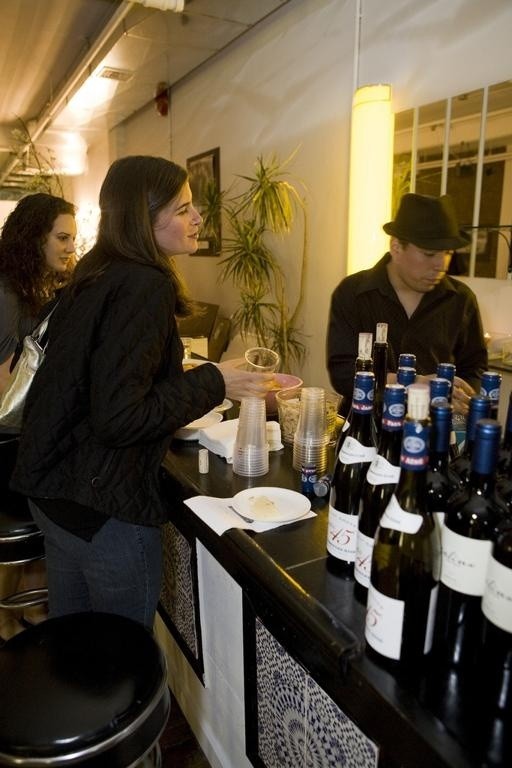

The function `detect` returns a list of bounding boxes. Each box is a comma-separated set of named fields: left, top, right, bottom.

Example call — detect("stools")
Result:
left=1, top=601, right=183, bottom=768
left=0, top=454, right=58, bottom=617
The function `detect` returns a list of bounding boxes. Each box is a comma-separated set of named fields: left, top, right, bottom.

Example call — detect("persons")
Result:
left=325, top=191, right=489, bottom=444
left=9, top=155, right=275, bottom=636
left=0, top=190, right=79, bottom=645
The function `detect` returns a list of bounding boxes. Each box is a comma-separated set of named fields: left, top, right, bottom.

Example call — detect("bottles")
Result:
left=472, top=529, right=511, bottom=670
left=354, top=383, right=410, bottom=604
left=436, top=419, right=509, bottom=665
left=362, top=384, right=442, bottom=671
left=325, top=371, right=377, bottom=580
left=321, top=322, right=511, bottom=507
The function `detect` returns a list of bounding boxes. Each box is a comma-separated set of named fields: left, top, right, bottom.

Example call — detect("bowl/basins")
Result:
left=275, top=388, right=340, bottom=447
left=263, top=374, right=305, bottom=418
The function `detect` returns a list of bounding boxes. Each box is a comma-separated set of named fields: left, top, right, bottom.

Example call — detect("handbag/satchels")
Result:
left=1, top=335, right=47, bottom=434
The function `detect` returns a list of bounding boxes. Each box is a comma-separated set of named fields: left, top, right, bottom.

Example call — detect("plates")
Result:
left=232, top=486, right=312, bottom=523
left=212, top=399, right=232, bottom=413
left=179, top=411, right=224, bottom=429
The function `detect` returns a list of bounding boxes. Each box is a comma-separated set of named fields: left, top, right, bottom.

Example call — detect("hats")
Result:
left=383, top=193, right=471, bottom=250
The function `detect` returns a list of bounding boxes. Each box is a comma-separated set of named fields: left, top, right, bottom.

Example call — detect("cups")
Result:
left=233, top=396, right=271, bottom=477
left=245, top=348, right=278, bottom=373
left=181, top=338, right=193, bottom=362
left=293, top=387, right=329, bottom=474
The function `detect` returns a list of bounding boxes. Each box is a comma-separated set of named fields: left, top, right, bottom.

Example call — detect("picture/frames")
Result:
left=183, top=146, right=221, bottom=258
